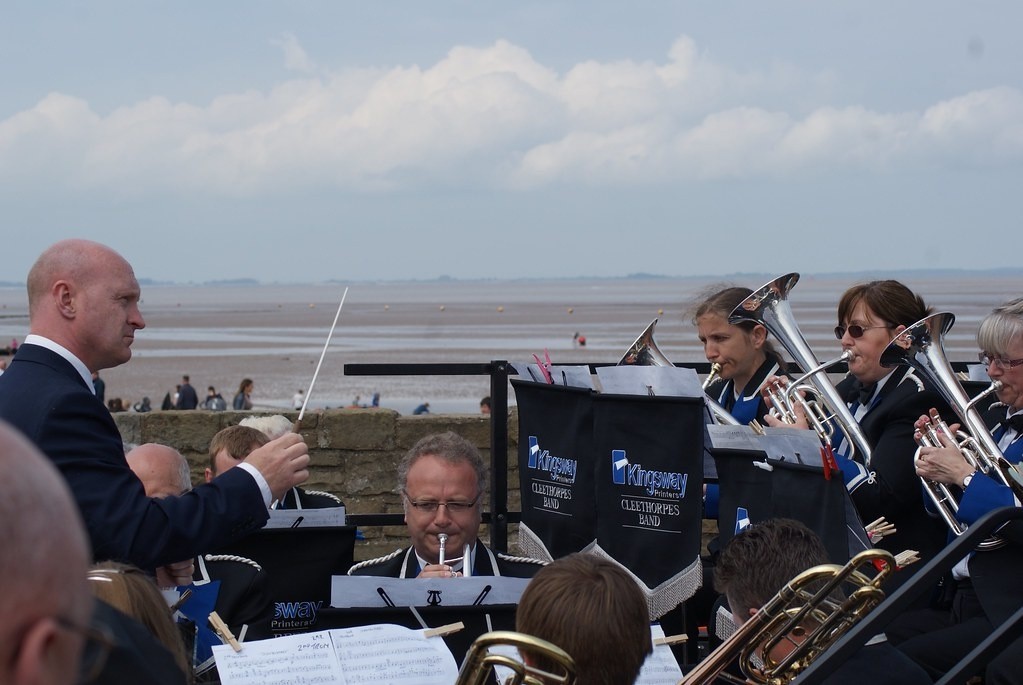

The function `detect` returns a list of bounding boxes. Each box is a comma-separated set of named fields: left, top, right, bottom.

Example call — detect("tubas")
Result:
left=679, top=547, right=899, bottom=685
left=616, top=316, right=743, bottom=428
left=455, top=631, right=578, bottom=685
left=878, top=310, right=1023, bottom=554
left=724, top=270, right=874, bottom=472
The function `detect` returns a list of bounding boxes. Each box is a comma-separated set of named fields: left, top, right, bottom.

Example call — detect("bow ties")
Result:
left=998, top=413, right=1023, bottom=432
left=847, top=380, right=878, bottom=406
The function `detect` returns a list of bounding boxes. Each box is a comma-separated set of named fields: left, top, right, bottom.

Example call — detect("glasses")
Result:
left=834, top=325, right=890, bottom=339
left=53, top=611, right=120, bottom=684
left=979, top=351, right=1023, bottom=370
left=403, top=488, right=483, bottom=512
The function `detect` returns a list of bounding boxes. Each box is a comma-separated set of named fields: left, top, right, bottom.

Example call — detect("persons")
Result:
left=87, top=595, right=188, bottom=685
left=761, top=280, right=947, bottom=624
left=514, top=554, right=652, bottom=685
left=572, top=332, right=578, bottom=342
left=469, top=397, right=491, bottom=414
left=239, top=414, right=347, bottom=514
left=204, top=424, right=271, bottom=482
left=713, top=517, right=935, bottom=685
left=0, top=420, right=89, bottom=685
left=372, top=393, right=379, bottom=406
left=886, top=297, right=1023, bottom=685
left=320, top=430, right=550, bottom=609
left=125, top=443, right=278, bottom=685
left=351, top=396, right=360, bottom=406
left=0, top=238, right=309, bottom=563
left=681, top=281, right=818, bottom=520
left=88, top=558, right=210, bottom=685
left=413, top=403, right=429, bottom=415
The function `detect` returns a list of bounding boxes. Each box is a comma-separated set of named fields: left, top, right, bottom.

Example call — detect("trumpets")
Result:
left=436, top=532, right=473, bottom=579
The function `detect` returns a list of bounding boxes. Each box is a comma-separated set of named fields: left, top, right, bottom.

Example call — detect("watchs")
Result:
left=962, top=469, right=977, bottom=491
